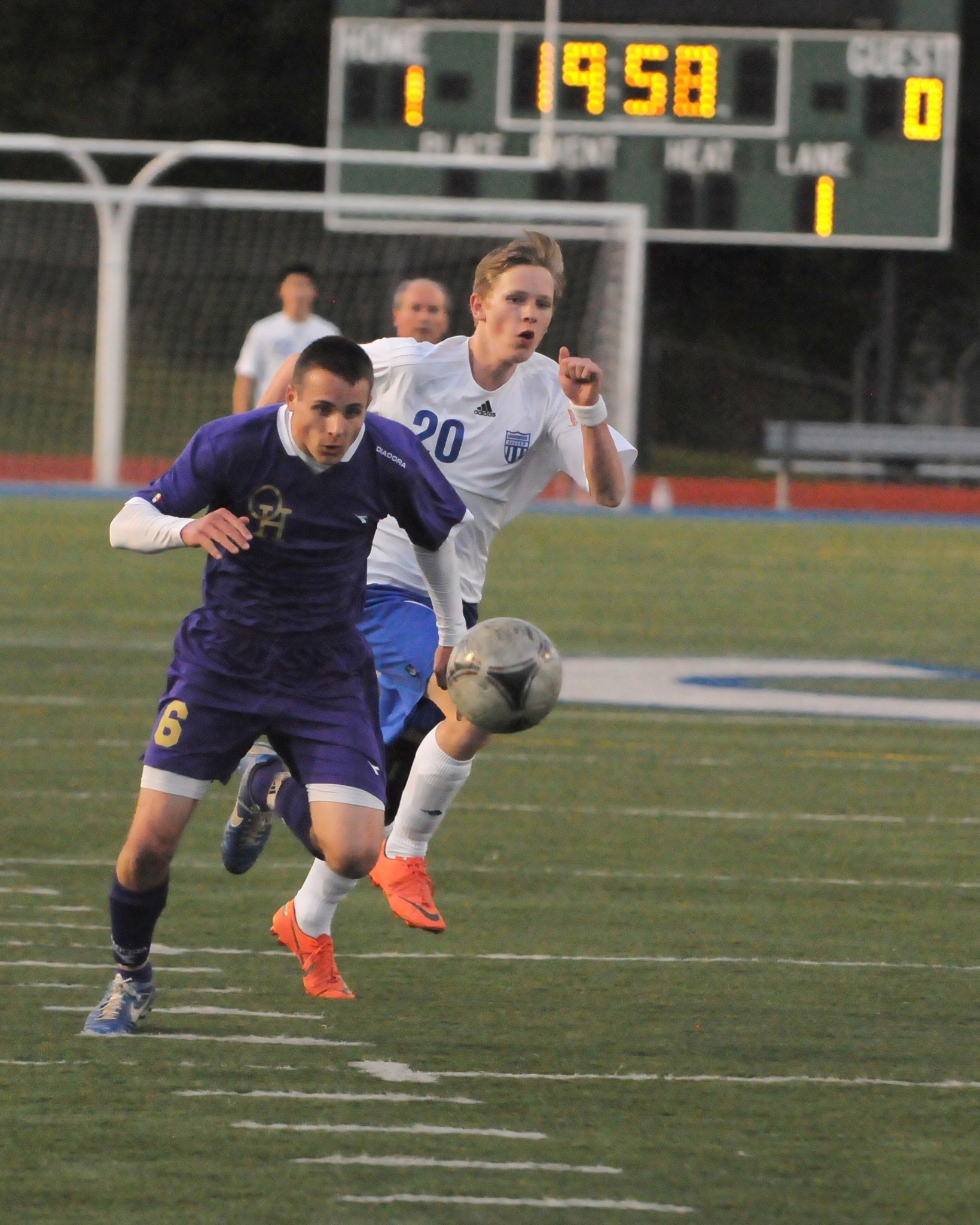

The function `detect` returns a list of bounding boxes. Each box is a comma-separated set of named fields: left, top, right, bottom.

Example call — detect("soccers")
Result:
left=447, top=618, right=563, bottom=733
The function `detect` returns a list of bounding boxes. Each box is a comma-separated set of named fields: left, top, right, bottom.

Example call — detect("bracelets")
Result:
left=571, top=394, right=607, bottom=426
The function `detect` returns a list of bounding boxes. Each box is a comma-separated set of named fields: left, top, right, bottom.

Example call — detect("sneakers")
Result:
left=221, top=742, right=283, bottom=874
left=81, top=974, right=156, bottom=1036
left=268, top=898, right=354, bottom=1001
left=368, top=836, right=448, bottom=931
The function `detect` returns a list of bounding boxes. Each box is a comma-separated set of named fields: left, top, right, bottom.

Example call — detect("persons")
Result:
left=393, top=279, right=449, bottom=344
left=84, top=336, right=468, bottom=1034
left=256, top=233, right=639, bottom=998
left=233, top=267, right=340, bottom=415
